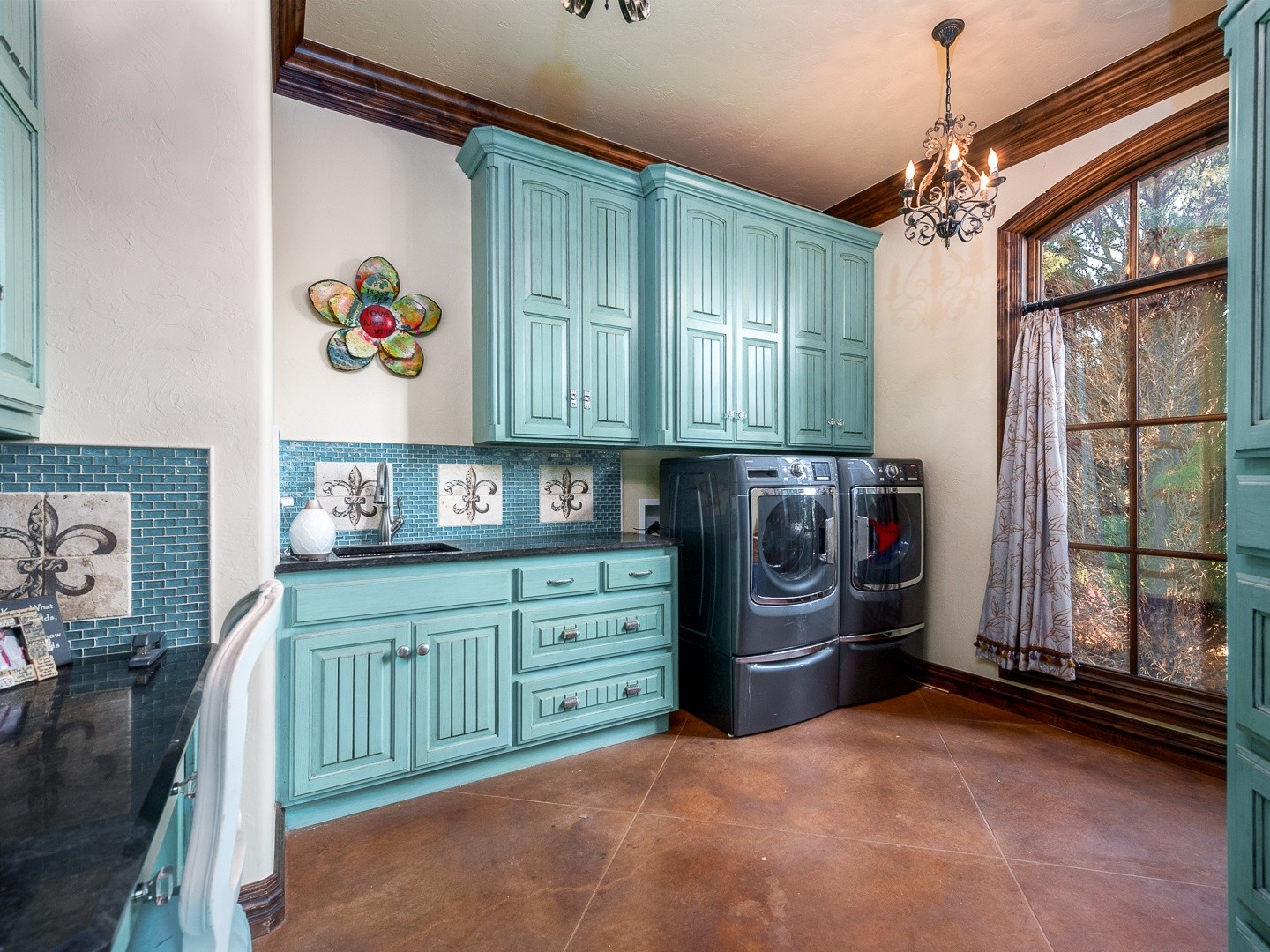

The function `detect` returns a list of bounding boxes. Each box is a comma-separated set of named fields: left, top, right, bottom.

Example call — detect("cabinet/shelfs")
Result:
left=279, top=545, right=680, bottom=831
left=452, top=124, right=647, bottom=448
left=639, top=163, right=787, bottom=448
left=787, top=202, right=883, bottom=456
left=1, top=642, right=217, bottom=952
left=0, top=0, right=44, bottom=437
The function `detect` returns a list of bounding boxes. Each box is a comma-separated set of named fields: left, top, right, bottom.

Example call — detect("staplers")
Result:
left=128, top=631, right=167, bottom=668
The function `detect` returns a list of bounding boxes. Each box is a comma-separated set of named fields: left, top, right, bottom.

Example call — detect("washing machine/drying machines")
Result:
left=828, top=455, right=927, bottom=710
left=657, top=451, right=842, bottom=739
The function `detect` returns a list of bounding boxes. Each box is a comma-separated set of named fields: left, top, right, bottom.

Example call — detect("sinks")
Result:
left=332, top=539, right=465, bottom=561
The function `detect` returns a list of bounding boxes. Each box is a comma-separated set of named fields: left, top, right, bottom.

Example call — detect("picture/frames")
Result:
left=1, top=608, right=58, bottom=691
left=0, top=678, right=59, bottom=758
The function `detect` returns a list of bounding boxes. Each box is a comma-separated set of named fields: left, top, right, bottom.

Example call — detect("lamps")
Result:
left=899, top=18, right=1007, bottom=250
left=562, top=0, right=651, bottom=24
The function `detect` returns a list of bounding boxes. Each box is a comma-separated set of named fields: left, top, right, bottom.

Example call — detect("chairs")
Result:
left=128, top=577, right=282, bottom=952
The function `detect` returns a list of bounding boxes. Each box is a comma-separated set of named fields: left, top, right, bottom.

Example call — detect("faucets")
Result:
left=373, top=461, right=405, bottom=547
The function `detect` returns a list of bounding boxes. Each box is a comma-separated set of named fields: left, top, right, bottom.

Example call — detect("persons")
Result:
left=0, top=628, right=26, bottom=667
left=0, top=703, right=25, bottom=742
